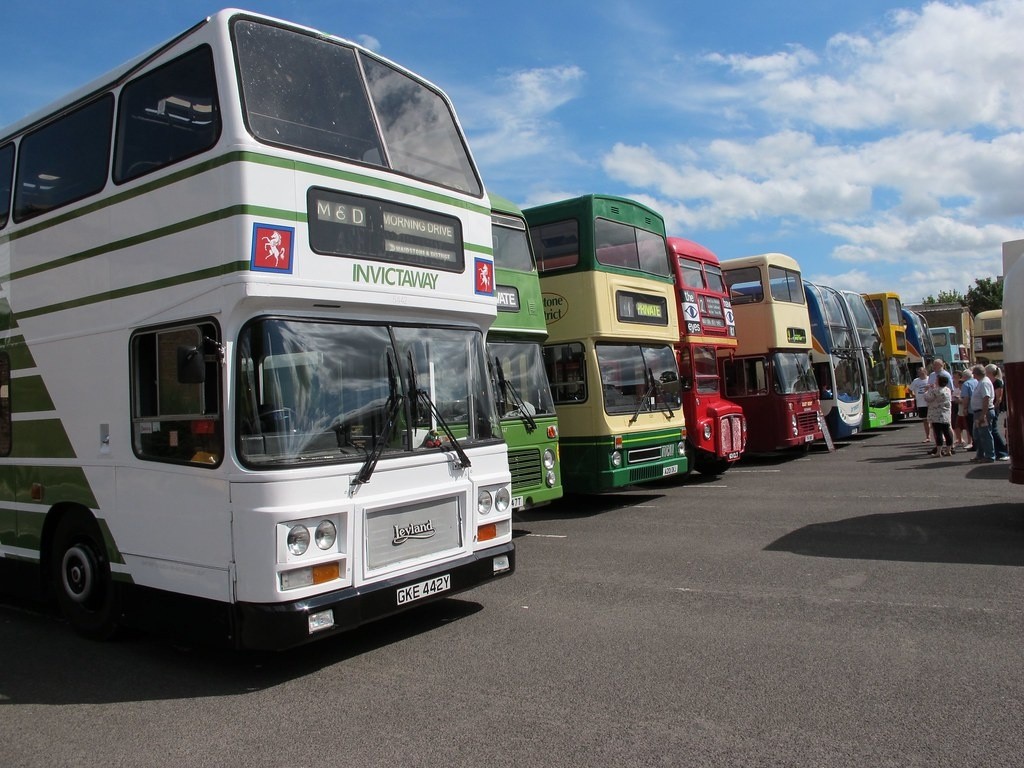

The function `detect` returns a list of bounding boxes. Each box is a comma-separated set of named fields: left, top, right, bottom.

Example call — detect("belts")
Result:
left=974, top=408, right=994, bottom=412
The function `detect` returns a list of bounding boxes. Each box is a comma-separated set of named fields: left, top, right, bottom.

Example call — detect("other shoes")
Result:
left=922, top=437, right=930, bottom=443
left=976, top=457, right=996, bottom=463
left=971, top=454, right=983, bottom=462
left=963, top=444, right=973, bottom=449
left=999, top=456, right=1011, bottom=460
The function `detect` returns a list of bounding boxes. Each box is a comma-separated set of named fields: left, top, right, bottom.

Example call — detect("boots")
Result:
left=931, top=446, right=942, bottom=458
left=943, top=446, right=951, bottom=455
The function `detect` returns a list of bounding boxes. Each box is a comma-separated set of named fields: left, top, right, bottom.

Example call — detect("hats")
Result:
left=972, top=364, right=986, bottom=375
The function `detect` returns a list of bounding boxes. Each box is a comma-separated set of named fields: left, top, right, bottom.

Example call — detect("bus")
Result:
left=521, top=193, right=689, bottom=492
left=731, top=278, right=877, bottom=441
left=242, top=191, right=564, bottom=512
left=2, top=7, right=516, bottom=645
left=900, top=306, right=936, bottom=382
left=860, top=292, right=916, bottom=417
left=537, top=238, right=747, bottom=477
left=929, top=326, right=963, bottom=377
left=974, top=308, right=1003, bottom=372
left=837, top=290, right=894, bottom=429
left=718, top=252, right=824, bottom=453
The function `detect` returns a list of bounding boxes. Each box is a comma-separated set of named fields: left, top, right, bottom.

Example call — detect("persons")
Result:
left=924, top=359, right=1010, bottom=460
left=907, top=366, right=932, bottom=443
left=970, top=364, right=995, bottom=463
left=923, top=374, right=954, bottom=457
